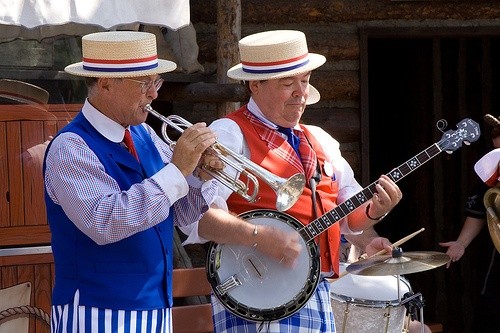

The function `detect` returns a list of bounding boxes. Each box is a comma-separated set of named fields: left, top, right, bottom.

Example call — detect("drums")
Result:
left=324, top=260, right=413, bottom=333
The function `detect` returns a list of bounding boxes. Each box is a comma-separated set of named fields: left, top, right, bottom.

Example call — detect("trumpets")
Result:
left=142, top=102, right=306, bottom=214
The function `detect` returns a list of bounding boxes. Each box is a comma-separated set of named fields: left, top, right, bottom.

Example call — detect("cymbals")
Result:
left=346, top=251, right=450, bottom=277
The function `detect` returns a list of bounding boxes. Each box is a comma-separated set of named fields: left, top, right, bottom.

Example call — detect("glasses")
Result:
left=121, top=78, right=164, bottom=94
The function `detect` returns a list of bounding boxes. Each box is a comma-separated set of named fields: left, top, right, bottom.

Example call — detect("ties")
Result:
left=278, top=126, right=300, bottom=155
left=124, top=130, right=140, bottom=163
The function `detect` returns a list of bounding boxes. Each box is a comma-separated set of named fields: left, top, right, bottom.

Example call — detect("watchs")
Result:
left=365, top=203, right=390, bottom=222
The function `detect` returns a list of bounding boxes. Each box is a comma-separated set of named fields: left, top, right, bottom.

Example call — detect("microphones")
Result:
left=483, top=114, right=500, bottom=130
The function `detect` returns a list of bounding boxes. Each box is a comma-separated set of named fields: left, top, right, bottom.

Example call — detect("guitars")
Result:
left=207, top=119, right=483, bottom=323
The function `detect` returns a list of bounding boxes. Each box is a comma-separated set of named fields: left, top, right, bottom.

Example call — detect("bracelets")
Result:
left=252, top=225, right=258, bottom=252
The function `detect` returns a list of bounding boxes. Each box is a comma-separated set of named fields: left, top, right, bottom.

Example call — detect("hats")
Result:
left=226, top=30, right=326, bottom=80
left=306, top=85, right=321, bottom=105
left=64, top=31, right=177, bottom=79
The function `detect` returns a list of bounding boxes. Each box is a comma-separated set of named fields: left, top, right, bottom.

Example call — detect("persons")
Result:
left=42, top=31, right=228, bottom=333
left=177, top=29, right=403, bottom=333
left=438, top=116, right=500, bottom=283
